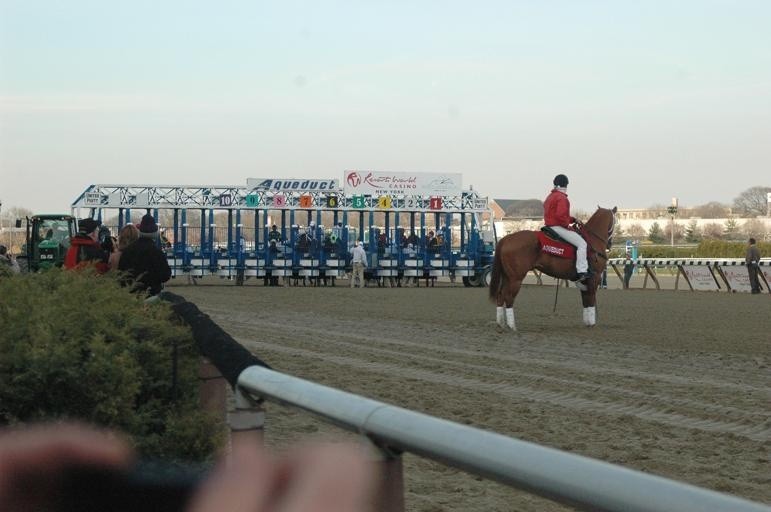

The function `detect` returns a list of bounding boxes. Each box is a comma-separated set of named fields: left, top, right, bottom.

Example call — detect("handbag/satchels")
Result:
left=137, top=242, right=170, bottom=296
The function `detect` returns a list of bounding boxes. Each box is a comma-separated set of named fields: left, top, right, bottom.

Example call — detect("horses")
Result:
left=264, top=233, right=438, bottom=289
left=487, top=203, right=619, bottom=333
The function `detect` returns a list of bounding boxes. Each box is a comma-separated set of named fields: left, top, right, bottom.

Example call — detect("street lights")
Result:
left=666, top=197, right=678, bottom=245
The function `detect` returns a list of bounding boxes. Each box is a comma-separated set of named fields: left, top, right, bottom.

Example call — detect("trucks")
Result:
left=15, top=213, right=76, bottom=272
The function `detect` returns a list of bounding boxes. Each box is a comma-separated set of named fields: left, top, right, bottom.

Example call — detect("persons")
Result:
left=0, top=424, right=388, bottom=512
left=543, top=174, right=594, bottom=286
left=624, top=255, right=634, bottom=289
left=0, top=213, right=171, bottom=301
left=268, top=222, right=446, bottom=289
left=745, top=238, right=761, bottom=294
left=598, top=259, right=609, bottom=289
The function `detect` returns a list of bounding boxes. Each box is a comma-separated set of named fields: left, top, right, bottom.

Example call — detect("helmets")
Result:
left=554, top=175, right=569, bottom=186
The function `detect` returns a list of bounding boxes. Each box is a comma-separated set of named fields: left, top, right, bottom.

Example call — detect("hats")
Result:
left=356, top=241, right=365, bottom=249
left=139, top=214, right=160, bottom=238
left=80, top=218, right=102, bottom=236
